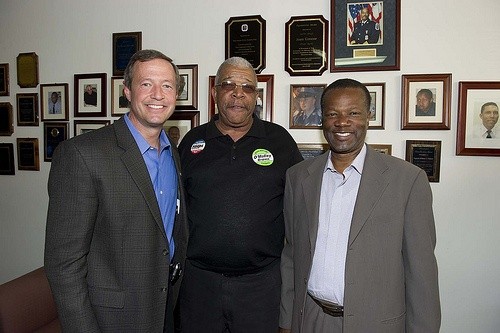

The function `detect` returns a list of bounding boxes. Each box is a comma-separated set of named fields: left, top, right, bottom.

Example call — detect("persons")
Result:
left=48, top=92, right=61, bottom=114
left=174, top=57, right=304, bottom=333
left=84, top=84, right=97, bottom=107
left=350, top=7, right=379, bottom=44
left=416, top=89, right=435, bottom=116
left=473, top=102, right=500, bottom=139
left=167, top=126, right=180, bottom=146
left=43, top=50, right=190, bottom=333
left=279, top=78, right=442, bottom=333
left=177, top=76, right=187, bottom=99
left=119, top=89, right=131, bottom=108
left=292, top=87, right=322, bottom=126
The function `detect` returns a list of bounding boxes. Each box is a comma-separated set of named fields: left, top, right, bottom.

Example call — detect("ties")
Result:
left=304, top=115, right=307, bottom=122
left=362, top=23, right=364, bottom=26
left=52, top=104, right=55, bottom=114
left=486, top=130, right=492, bottom=138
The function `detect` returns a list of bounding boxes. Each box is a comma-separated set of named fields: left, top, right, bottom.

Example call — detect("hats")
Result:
left=297, top=87, right=320, bottom=98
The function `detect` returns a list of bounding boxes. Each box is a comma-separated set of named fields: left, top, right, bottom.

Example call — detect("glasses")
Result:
left=215, top=80, right=257, bottom=93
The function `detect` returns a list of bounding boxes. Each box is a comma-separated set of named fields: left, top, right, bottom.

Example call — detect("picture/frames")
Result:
left=16, top=138, right=41, bottom=171
left=289, top=82, right=327, bottom=130
left=40, top=82, right=70, bottom=121
left=43, top=122, right=69, bottom=163
left=110, top=77, right=131, bottom=117
left=16, top=52, right=40, bottom=89
left=361, top=82, right=386, bottom=130
left=327, top=0, right=401, bottom=73
left=174, top=64, right=199, bottom=110
left=15, top=93, right=40, bottom=127
left=72, top=72, right=107, bottom=118
left=0, top=143, right=16, bottom=176
left=0, top=62, right=10, bottom=96
left=400, top=73, right=452, bottom=131
left=208, top=73, right=275, bottom=124
left=162, top=110, right=200, bottom=151
left=73, top=119, right=110, bottom=140
left=0, top=101, right=14, bottom=136
left=455, top=80, right=500, bottom=157
left=110, top=32, right=142, bottom=77
left=367, top=144, right=392, bottom=156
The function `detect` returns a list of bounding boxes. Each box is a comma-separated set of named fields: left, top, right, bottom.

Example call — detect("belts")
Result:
left=307, top=292, right=344, bottom=317
left=169, top=261, right=183, bottom=286
